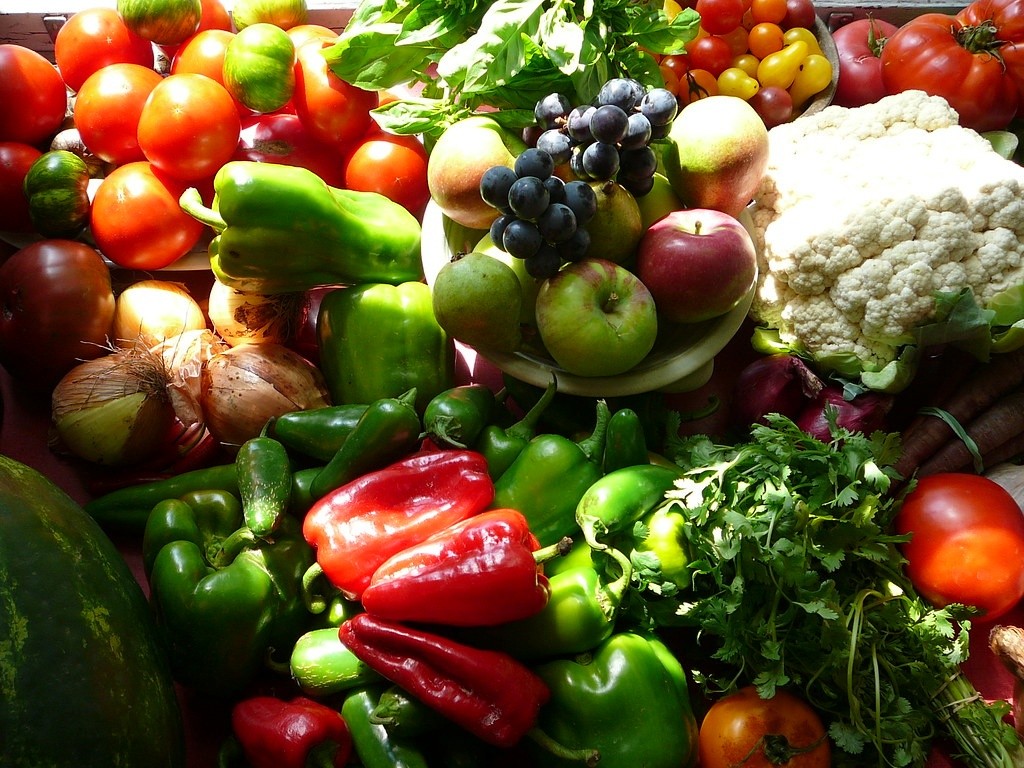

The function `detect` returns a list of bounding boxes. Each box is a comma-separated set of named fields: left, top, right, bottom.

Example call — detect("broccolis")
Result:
left=736, top=87, right=1024, bottom=394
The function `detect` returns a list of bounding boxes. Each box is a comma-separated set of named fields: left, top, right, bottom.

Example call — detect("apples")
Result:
left=426, top=114, right=757, bottom=377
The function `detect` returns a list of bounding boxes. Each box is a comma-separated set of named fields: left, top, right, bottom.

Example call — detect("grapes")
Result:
left=479, top=80, right=676, bottom=283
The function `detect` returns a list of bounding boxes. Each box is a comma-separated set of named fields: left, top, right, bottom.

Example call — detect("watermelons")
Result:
left=0, top=457, right=187, bottom=768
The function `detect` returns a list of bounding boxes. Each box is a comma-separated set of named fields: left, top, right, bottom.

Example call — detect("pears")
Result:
left=433, top=250, right=527, bottom=357
left=666, top=92, right=770, bottom=221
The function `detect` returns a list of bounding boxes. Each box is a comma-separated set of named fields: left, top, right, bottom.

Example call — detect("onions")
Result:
left=45, top=272, right=328, bottom=467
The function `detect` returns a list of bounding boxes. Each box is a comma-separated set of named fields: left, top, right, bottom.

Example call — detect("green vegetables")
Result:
left=323, top=0, right=701, bottom=144
left=613, top=395, right=1024, bottom=768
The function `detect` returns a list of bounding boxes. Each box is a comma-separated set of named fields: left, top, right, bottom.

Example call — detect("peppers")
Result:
left=316, top=279, right=454, bottom=411
left=74, top=370, right=701, bottom=768
left=180, top=158, right=422, bottom=292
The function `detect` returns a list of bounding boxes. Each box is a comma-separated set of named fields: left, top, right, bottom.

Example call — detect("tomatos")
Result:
left=635, top=0, right=1024, bottom=136
left=0, top=0, right=429, bottom=381
left=697, top=685, right=830, bottom=768
left=896, top=470, right=1024, bottom=620
